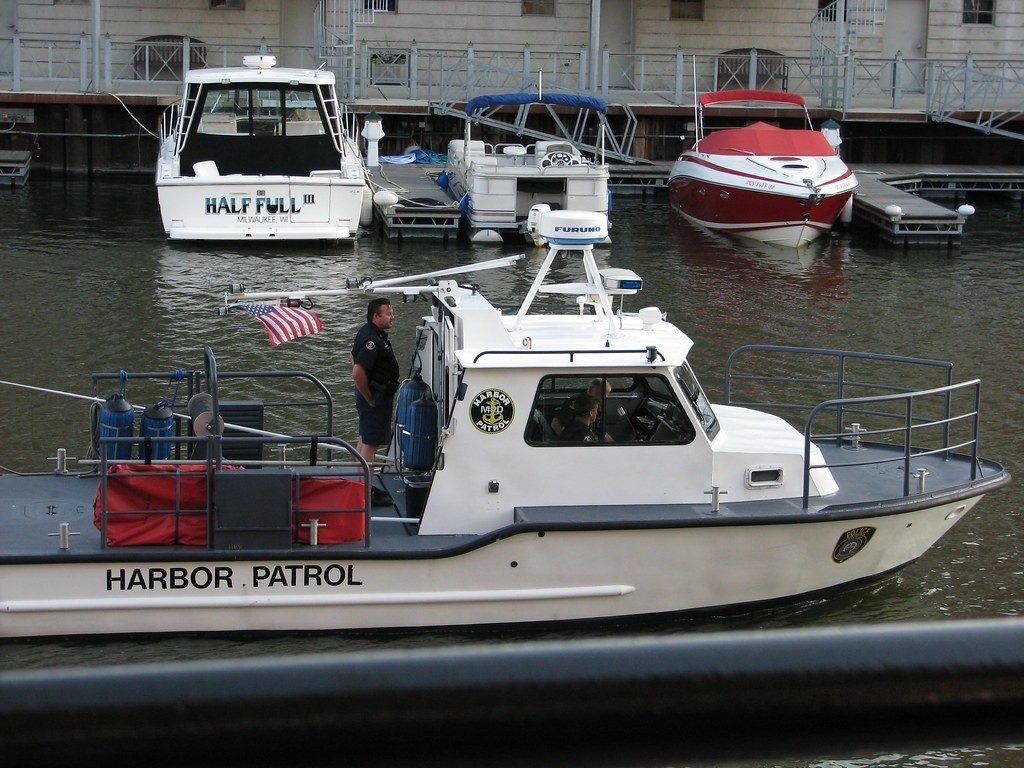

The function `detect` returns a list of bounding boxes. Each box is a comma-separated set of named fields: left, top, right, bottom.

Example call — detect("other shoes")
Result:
left=371, top=486, right=394, bottom=506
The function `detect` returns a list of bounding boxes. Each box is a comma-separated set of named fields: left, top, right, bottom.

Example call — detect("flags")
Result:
left=230, top=302, right=323, bottom=349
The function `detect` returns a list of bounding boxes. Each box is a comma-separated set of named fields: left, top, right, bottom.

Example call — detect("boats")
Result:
left=155, top=55, right=373, bottom=247
left=1, top=203, right=1011, bottom=646
left=437, top=68, right=614, bottom=246
left=666, top=55, right=860, bottom=250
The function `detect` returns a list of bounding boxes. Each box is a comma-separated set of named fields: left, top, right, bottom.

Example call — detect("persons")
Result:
left=550, top=377, right=612, bottom=443
left=350, top=299, right=400, bottom=507
